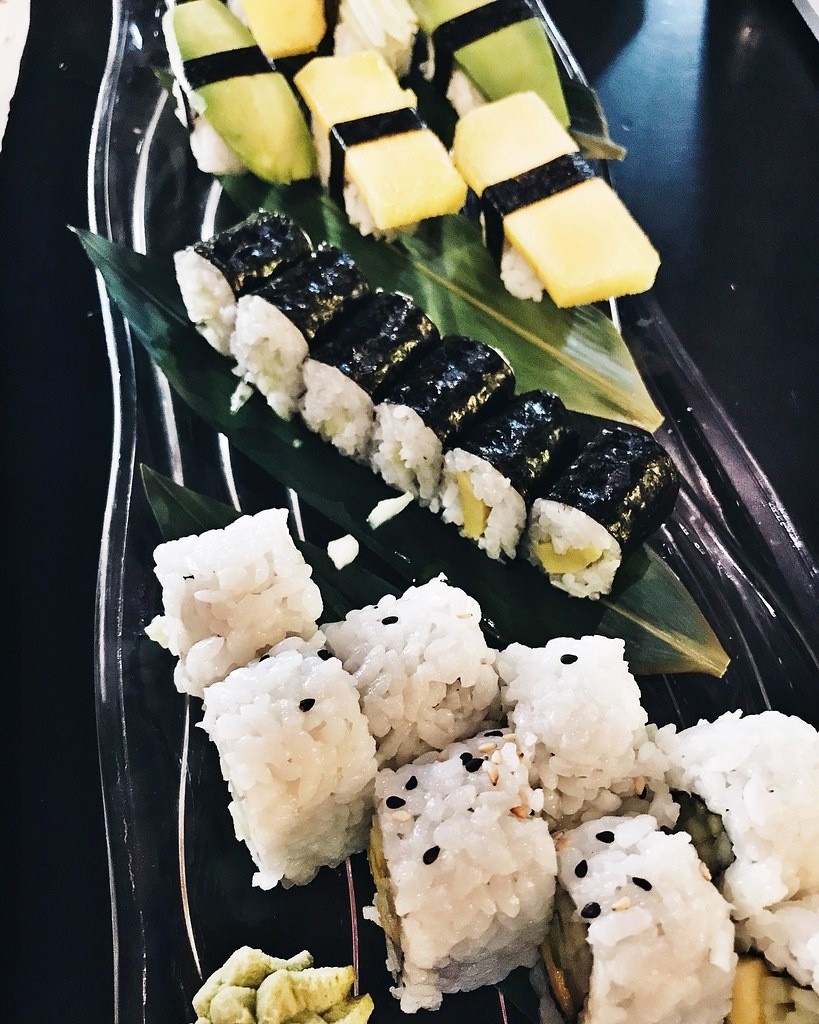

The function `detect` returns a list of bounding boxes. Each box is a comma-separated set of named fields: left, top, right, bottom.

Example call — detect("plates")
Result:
left=87, top=1, right=819, bottom=1022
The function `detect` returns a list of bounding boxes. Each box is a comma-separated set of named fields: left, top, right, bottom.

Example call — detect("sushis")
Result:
left=138, top=0, right=819, bottom=1024
